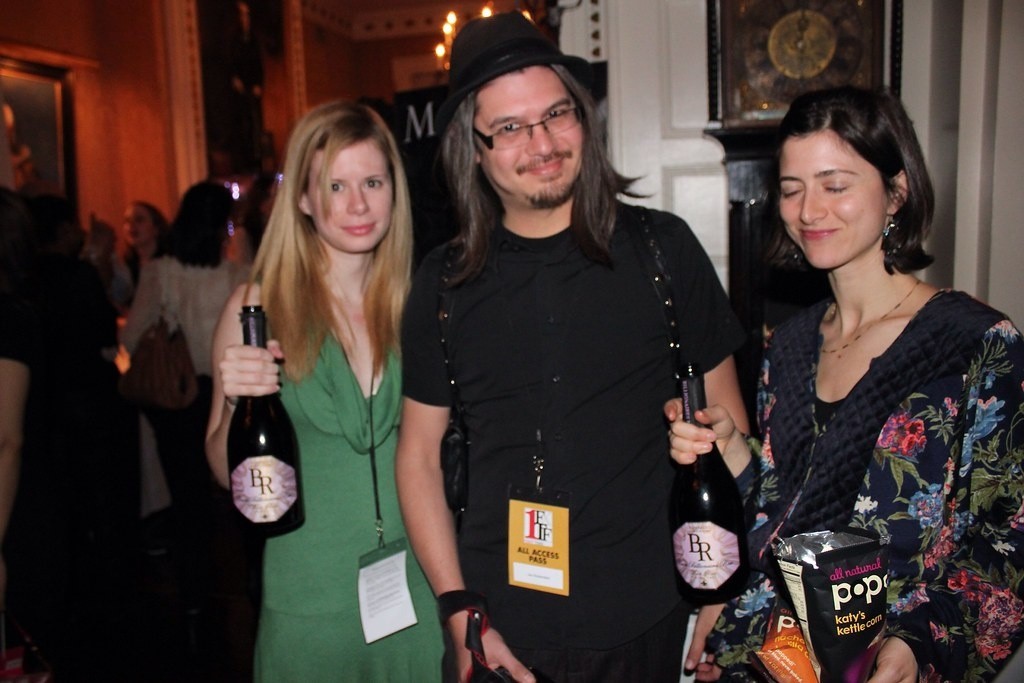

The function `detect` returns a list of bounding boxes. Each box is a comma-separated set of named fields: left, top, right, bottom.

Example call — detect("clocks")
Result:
left=702, top=1, right=902, bottom=437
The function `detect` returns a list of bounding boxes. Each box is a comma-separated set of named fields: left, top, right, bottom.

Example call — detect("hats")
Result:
left=432, top=10, right=608, bottom=136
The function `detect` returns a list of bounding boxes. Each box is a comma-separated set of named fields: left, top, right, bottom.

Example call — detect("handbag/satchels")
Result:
left=119, top=254, right=197, bottom=409
left=0, top=608, right=55, bottom=683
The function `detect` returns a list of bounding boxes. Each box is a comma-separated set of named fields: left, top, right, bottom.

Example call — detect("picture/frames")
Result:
left=0, top=54, right=77, bottom=220
left=181, top=0, right=307, bottom=189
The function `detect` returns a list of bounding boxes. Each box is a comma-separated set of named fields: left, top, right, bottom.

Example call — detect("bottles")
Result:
left=672, top=361, right=751, bottom=605
left=226, top=304, right=305, bottom=540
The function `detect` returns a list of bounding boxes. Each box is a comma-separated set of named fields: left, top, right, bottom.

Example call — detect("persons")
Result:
left=0, top=11, right=1024, bottom=683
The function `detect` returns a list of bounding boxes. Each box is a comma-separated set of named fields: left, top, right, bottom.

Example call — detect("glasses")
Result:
left=472, top=106, right=582, bottom=151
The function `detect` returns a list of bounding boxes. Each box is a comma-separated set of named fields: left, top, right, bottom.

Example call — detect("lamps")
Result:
left=433, top=7, right=535, bottom=72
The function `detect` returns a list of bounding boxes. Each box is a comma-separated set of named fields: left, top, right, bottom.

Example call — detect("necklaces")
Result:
left=821, top=280, right=922, bottom=353
